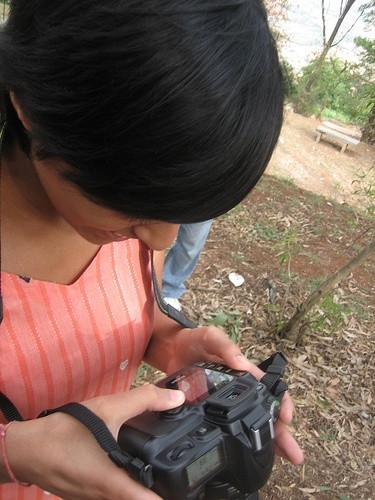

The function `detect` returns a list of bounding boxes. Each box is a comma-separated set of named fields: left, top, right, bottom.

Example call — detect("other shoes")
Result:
left=163, top=297, right=182, bottom=311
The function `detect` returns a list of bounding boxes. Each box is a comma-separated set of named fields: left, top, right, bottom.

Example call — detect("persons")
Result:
left=161, top=219, right=214, bottom=312
left=0, top=0, right=304, bottom=500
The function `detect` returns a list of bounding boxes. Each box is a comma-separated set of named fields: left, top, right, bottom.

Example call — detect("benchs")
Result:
left=316, top=122, right=360, bottom=152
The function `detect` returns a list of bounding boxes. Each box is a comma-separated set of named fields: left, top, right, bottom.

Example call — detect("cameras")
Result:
left=110, top=359, right=279, bottom=499
